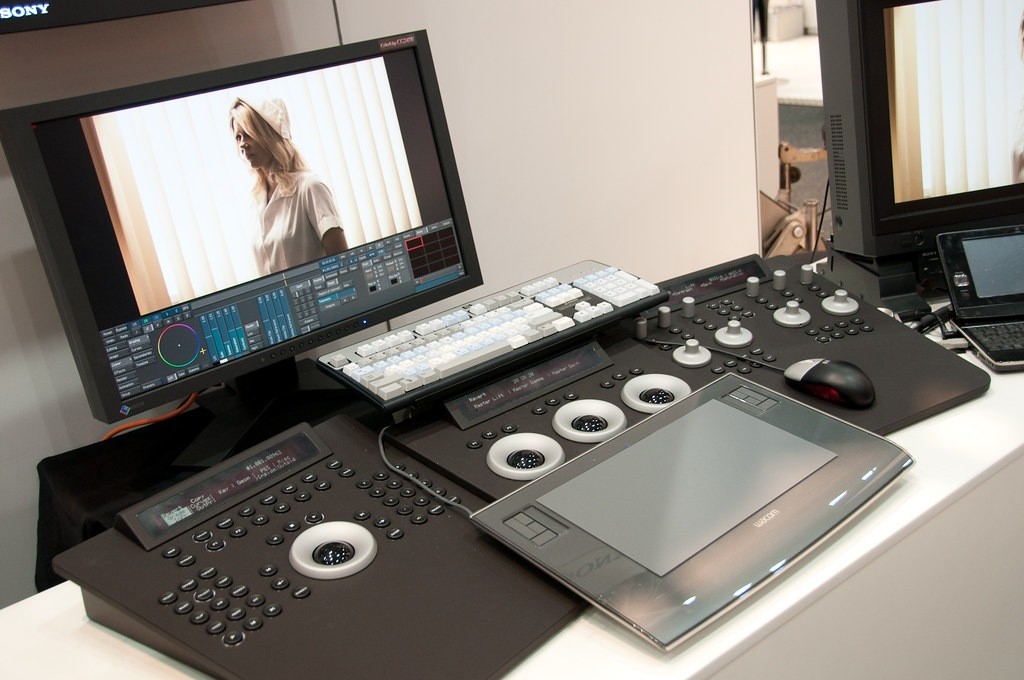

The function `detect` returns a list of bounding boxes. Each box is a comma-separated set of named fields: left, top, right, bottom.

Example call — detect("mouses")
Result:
left=783, top=358, right=876, bottom=410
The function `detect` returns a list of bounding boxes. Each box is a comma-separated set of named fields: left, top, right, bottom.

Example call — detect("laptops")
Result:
left=935, top=225, right=1024, bottom=372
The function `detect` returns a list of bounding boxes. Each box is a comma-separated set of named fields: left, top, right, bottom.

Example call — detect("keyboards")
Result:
left=316, top=259, right=670, bottom=414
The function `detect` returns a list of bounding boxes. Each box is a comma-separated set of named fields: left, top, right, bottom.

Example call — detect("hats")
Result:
left=236, top=95, right=291, bottom=140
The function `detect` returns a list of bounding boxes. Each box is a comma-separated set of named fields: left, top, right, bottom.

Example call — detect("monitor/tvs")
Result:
left=812, top=0, right=1024, bottom=251
left=0, top=27, right=482, bottom=466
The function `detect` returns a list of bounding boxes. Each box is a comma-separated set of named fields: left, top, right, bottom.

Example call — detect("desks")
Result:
left=0, top=256, right=1024, bottom=679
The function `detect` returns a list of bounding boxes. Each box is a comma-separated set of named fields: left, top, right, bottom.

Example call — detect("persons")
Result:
left=228, top=95, right=348, bottom=279
